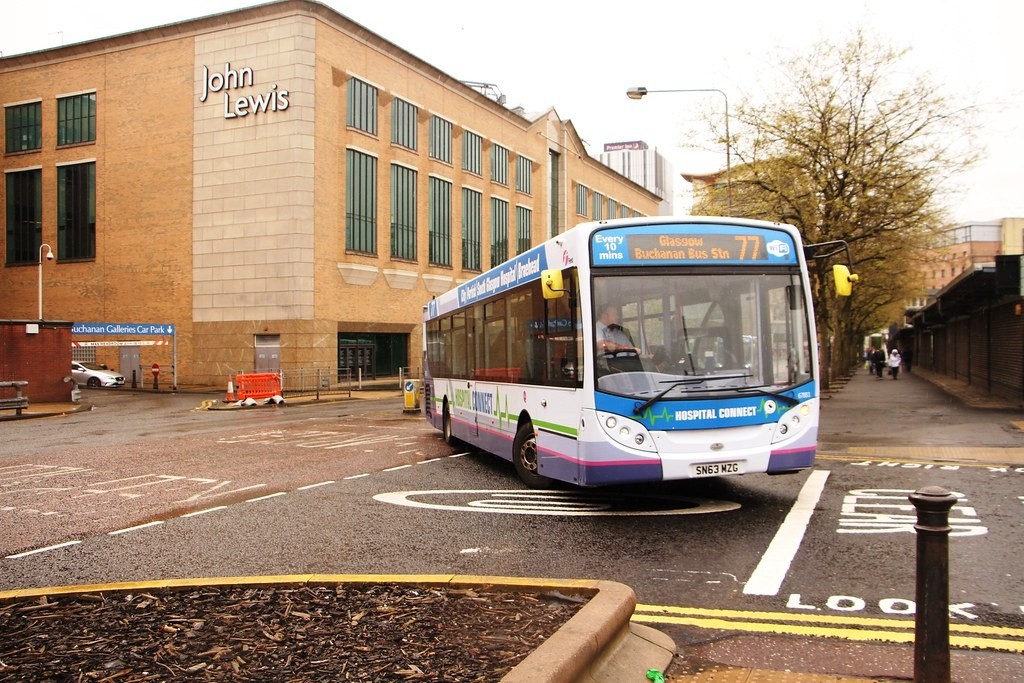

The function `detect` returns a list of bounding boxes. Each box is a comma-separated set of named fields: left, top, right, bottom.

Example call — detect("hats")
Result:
left=892, top=349, right=898, bottom=354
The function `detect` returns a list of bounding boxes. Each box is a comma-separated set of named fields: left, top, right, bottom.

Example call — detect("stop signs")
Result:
left=151, top=364, right=160, bottom=376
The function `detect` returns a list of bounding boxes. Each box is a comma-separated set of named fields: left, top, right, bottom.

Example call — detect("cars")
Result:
left=71, top=361, right=125, bottom=387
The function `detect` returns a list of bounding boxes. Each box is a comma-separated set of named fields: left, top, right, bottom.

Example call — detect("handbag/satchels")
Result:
left=887, top=368, right=893, bottom=375
left=881, top=361, right=886, bottom=367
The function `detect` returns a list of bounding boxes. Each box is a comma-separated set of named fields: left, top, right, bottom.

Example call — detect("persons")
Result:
left=863, top=346, right=886, bottom=380
left=903, top=346, right=914, bottom=371
left=595, top=304, right=643, bottom=372
left=888, top=349, right=901, bottom=380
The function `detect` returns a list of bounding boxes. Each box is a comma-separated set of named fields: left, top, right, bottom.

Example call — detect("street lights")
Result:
left=628, top=86, right=732, bottom=216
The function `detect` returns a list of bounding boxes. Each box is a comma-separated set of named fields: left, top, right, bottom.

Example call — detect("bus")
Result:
left=420, top=215, right=859, bottom=488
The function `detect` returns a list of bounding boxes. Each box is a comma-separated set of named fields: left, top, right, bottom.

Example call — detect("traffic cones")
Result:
left=223, top=374, right=238, bottom=403
